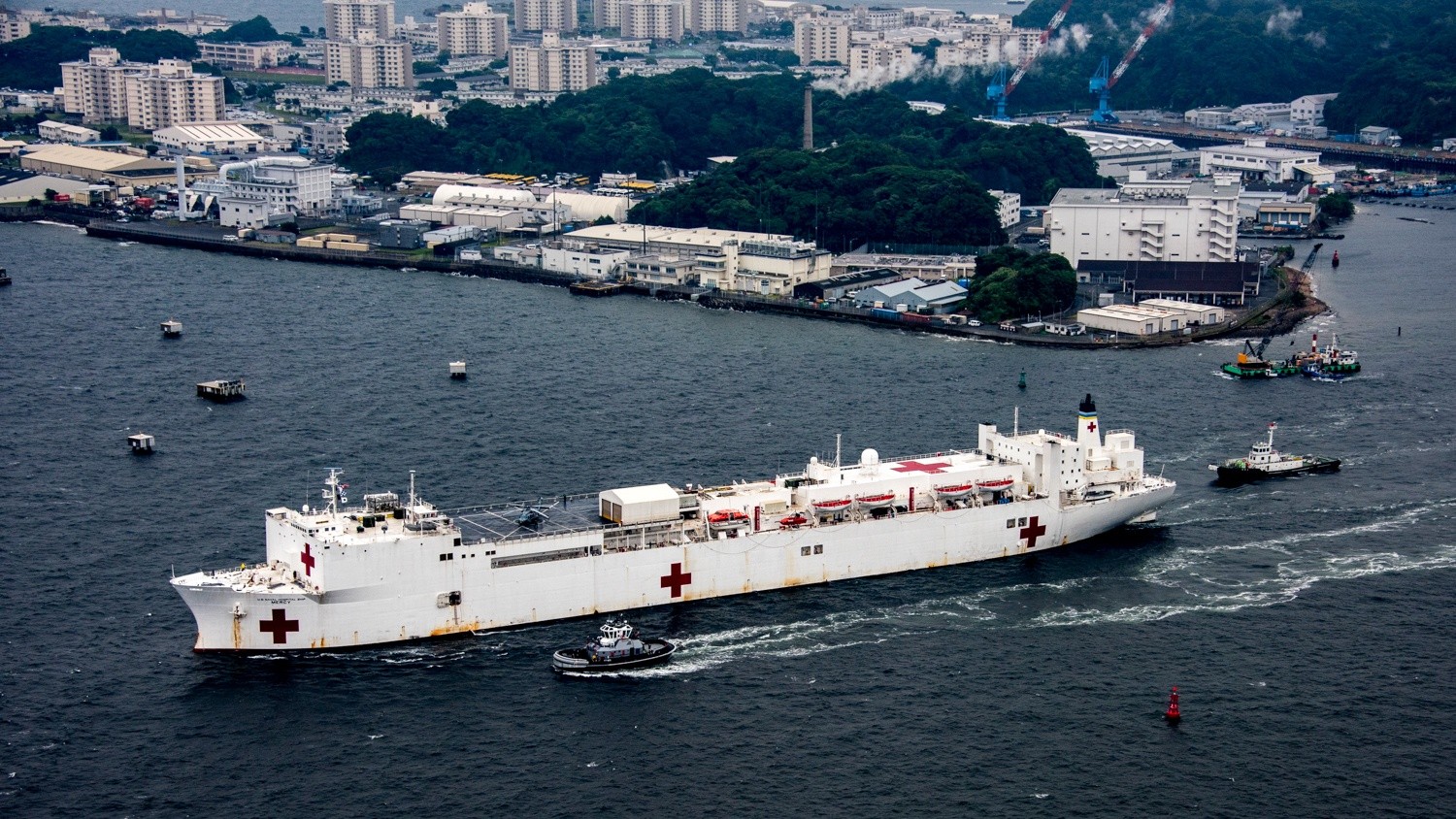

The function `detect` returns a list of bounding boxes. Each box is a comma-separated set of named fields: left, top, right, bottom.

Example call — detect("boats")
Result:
left=934, top=484, right=972, bottom=497
left=975, top=479, right=1014, bottom=492
left=778, top=516, right=807, bottom=525
left=1215, top=422, right=1342, bottom=486
left=1220, top=332, right=1362, bottom=381
left=551, top=611, right=677, bottom=673
left=1333, top=255, right=1340, bottom=265
left=855, top=493, right=896, bottom=509
left=707, top=509, right=750, bottom=531
left=812, top=500, right=852, bottom=513
left=170, top=393, right=1177, bottom=674
left=1084, top=490, right=1116, bottom=502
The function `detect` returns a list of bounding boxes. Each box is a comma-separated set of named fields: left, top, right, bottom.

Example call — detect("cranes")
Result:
left=1087, top=0, right=1173, bottom=123
left=1242, top=242, right=1324, bottom=361
left=986, top=0, right=1072, bottom=123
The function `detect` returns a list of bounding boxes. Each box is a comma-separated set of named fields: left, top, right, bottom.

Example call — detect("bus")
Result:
left=484, top=173, right=537, bottom=187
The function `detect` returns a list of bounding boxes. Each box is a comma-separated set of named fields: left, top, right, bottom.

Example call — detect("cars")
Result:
left=386, top=192, right=433, bottom=203
left=540, top=172, right=590, bottom=190
left=1392, top=142, right=1401, bottom=148
left=968, top=319, right=984, bottom=327
